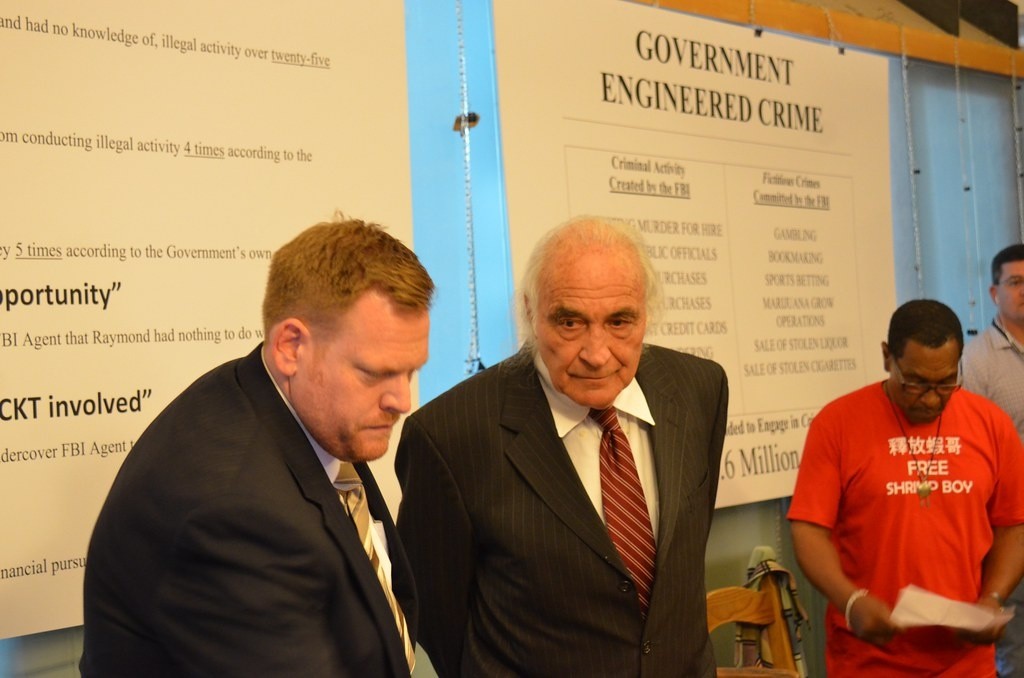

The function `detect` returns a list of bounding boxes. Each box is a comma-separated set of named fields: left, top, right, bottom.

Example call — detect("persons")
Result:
left=955, top=241, right=1024, bottom=678
left=393, top=211, right=731, bottom=678
left=78, top=209, right=438, bottom=678
left=785, top=297, right=1024, bottom=678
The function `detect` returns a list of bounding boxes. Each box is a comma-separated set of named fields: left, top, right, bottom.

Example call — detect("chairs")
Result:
left=707, top=574, right=801, bottom=678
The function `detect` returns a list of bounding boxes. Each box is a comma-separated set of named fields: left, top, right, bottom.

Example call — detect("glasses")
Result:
left=894, top=359, right=964, bottom=394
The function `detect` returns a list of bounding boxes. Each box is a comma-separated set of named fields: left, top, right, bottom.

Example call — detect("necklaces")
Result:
left=882, top=378, right=943, bottom=511
left=991, top=318, right=1023, bottom=359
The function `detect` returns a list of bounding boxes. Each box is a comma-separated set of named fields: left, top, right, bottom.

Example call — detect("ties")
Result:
left=334, top=462, right=417, bottom=675
left=588, top=406, right=658, bottom=620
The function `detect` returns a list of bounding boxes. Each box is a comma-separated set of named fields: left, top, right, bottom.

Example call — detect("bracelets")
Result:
left=840, top=589, right=869, bottom=633
left=979, top=590, right=1005, bottom=606
